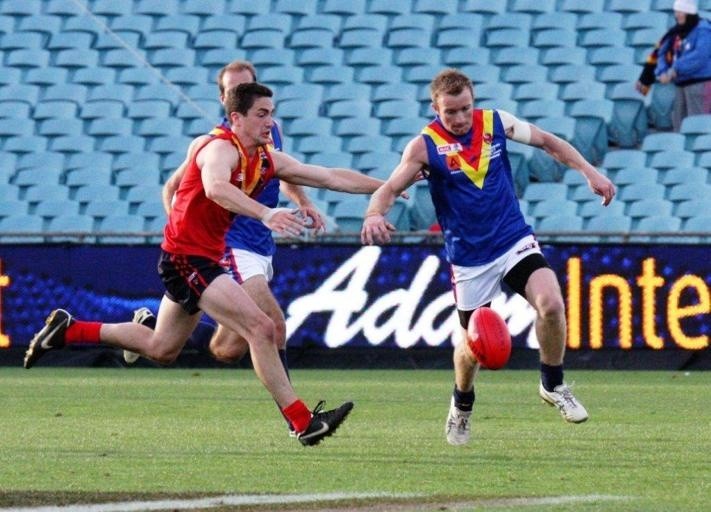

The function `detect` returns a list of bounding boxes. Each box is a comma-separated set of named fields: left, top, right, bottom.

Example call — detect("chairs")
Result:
left=1, top=0, right=711, bottom=242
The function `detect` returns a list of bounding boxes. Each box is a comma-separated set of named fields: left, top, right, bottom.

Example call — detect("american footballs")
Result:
left=468, top=307, right=511, bottom=369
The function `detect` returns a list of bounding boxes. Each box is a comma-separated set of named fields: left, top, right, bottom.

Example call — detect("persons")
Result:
left=360, top=70, right=620, bottom=448
left=635, top=0, right=711, bottom=129
left=122, top=59, right=330, bottom=440
left=22, top=81, right=426, bottom=448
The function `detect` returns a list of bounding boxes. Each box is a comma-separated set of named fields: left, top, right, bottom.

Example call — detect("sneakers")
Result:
left=123, top=307, right=156, bottom=364
left=289, top=401, right=353, bottom=446
left=445, top=391, right=474, bottom=448
left=538, top=376, right=589, bottom=423
left=24, top=308, right=75, bottom=370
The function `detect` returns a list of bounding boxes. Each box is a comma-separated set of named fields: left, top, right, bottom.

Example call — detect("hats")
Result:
left=672, top=0, right=700, bottom=16
